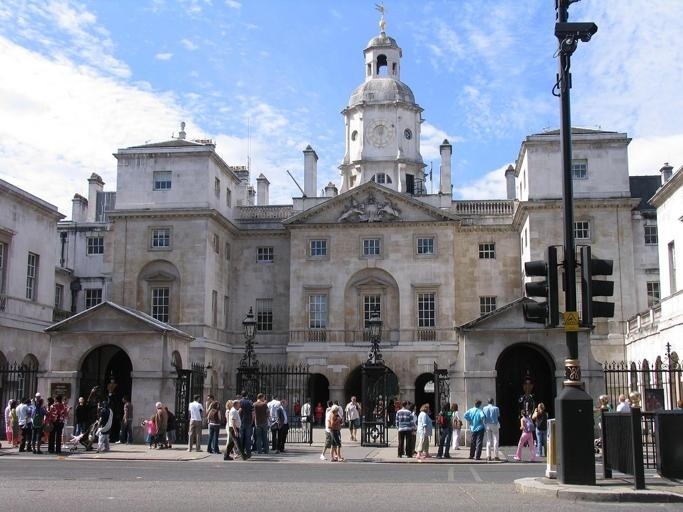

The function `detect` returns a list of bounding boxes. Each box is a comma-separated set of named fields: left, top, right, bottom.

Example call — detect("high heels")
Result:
left=207, top=448, right=222, bottom=454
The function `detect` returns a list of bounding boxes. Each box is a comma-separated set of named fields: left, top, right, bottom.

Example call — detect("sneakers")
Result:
left=397, top=451, right=451, bottom=459
left=320, top=455, right=345, bottom=462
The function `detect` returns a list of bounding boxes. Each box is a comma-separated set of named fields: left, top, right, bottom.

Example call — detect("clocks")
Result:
left=366, top=119, right=394, bottom=148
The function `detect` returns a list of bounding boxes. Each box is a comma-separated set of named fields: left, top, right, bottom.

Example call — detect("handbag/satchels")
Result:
left=453, top=416, right=462, bottom=429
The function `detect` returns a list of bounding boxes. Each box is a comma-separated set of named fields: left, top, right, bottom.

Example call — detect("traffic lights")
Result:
left=520, top=242, right=560, bottom=332
left=579, top=242, right=614, bottom=330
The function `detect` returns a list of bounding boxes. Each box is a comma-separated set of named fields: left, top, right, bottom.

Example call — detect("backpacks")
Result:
left=33, top=410, right=42, bottom=428
left=524, top=417, right=534, bottom=432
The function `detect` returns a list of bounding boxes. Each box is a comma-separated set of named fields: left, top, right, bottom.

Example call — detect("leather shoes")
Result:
left=224, top=451, right=252, bottom=460
left=19, top=448, right=61, bottom=454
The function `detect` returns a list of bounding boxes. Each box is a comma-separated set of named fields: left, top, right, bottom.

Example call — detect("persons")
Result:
left=616, top=394, right=631, bottom=411
left=3, top=379, right=134, bottom=454
left=515, top=402, right=547, bottom=462
left=375, top=394, right=501, bottom=463
left=140, top=396, right=361, bottom=464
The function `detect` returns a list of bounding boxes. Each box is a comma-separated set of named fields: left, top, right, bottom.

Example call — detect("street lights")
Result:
left=234, top=304, right=261, bottom=400
left=359, top=304, right=391, bottom=449
